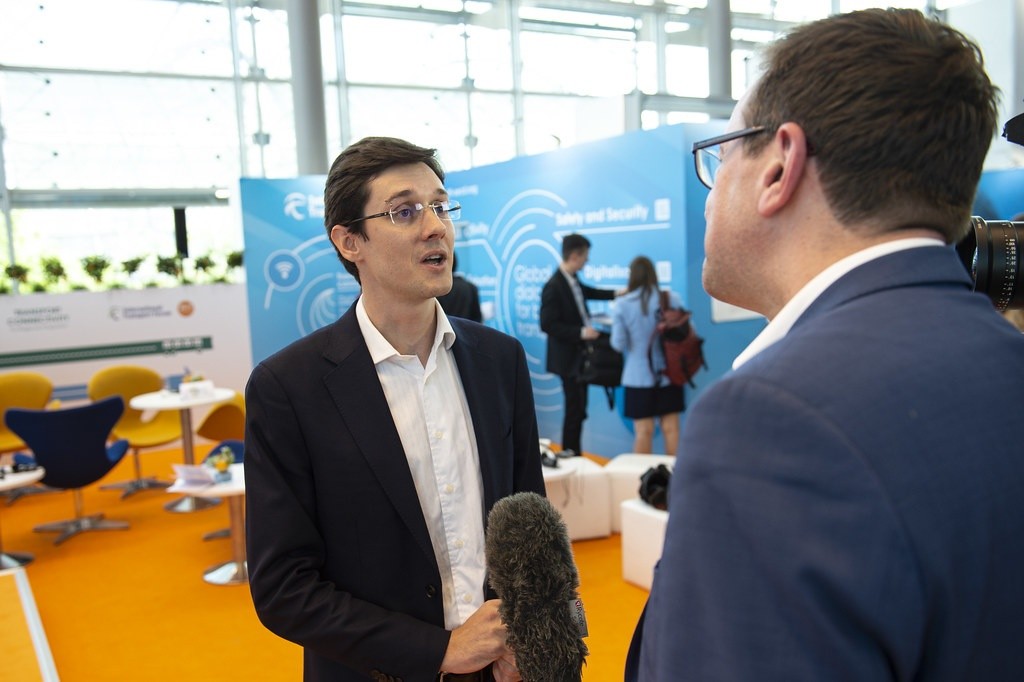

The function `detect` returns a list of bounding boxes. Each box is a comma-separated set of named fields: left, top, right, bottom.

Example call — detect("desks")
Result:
left=129, top=386, right=236, bottom=513
left=175, top=462, right=249, bottom=586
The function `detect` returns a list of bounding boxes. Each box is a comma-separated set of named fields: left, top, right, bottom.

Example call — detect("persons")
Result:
left=624, top=6, right=1024, bottom=682
left=609, top=255, right=687, bottom=456
left=244, top=137, right=548, bottom=682
left=435, top=250, right=483, bottom=324
left=538, top=233, right=629, bottom=457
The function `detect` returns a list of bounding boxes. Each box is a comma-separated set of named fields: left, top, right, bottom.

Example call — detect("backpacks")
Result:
left=647, top=291, right=708, bottom=388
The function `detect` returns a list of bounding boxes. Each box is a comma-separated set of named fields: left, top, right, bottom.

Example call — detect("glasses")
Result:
left=344, top=199, right=462, bottom=227
left=692, top=125, right=816, bottom=189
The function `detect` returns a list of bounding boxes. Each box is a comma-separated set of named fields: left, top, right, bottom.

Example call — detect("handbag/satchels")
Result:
left=582, top=331, right=623, bottom=411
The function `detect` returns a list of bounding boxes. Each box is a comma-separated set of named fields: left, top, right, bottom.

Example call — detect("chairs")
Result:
left=4, top=395, right=130, bottom=547
left=88, top=365, right=182, bottom=501
left=0, top=370, right=62, bottom=504
left=198, top=392, right=245, bottom=540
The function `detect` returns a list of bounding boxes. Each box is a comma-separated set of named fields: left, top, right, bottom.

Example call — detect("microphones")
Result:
left=485, top=490, right=588, bottom=682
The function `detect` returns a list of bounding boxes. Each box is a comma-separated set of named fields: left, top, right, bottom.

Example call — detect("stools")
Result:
left=549, top=452, right=676, bottom=592
